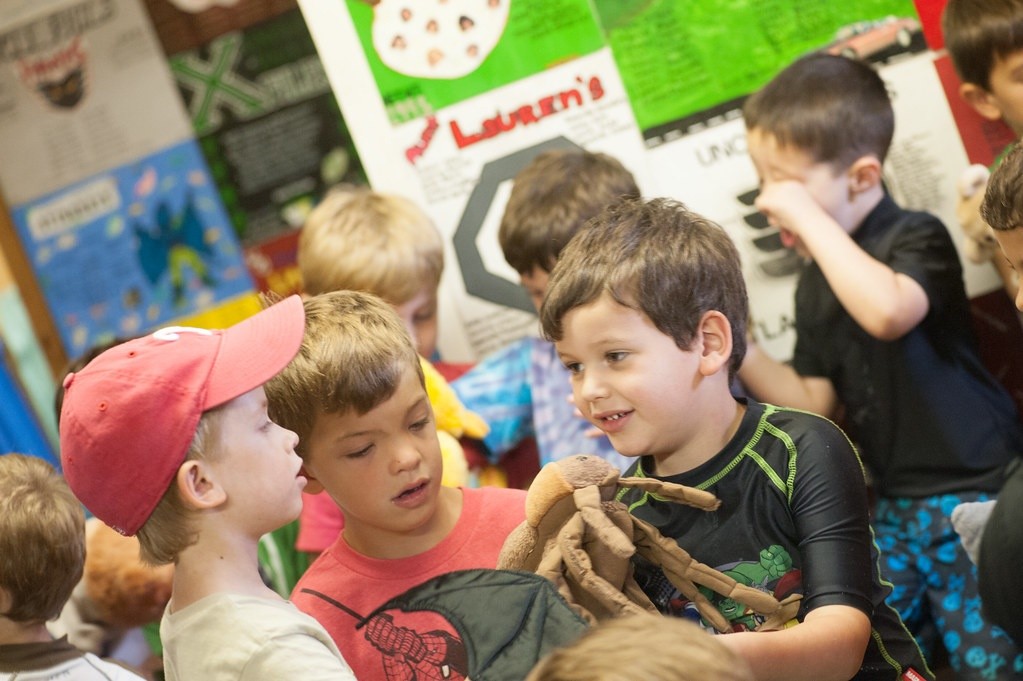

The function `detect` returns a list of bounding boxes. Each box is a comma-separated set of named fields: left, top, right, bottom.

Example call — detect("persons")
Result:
left=0, top=0, right=1022, bottom=680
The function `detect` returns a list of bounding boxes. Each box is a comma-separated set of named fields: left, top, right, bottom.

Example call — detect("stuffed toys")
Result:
left=496, top=453, right=802, bottom=634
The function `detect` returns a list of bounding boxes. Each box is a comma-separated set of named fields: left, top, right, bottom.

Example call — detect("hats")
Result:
left=61, top=292, right=306, bottom=536
